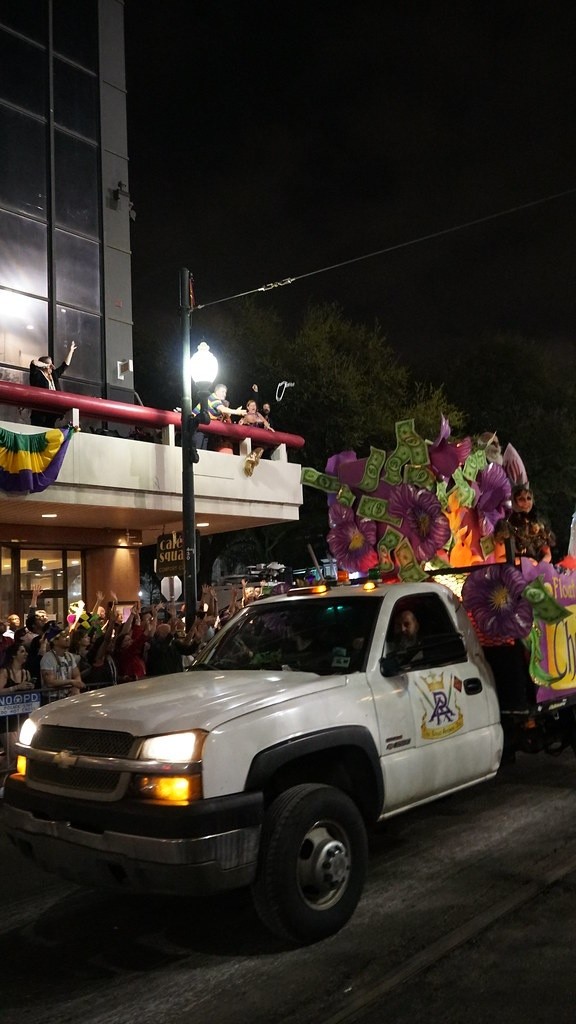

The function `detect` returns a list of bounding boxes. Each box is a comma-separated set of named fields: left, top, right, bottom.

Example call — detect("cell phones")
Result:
left=29, top=677, right=37, bottom=684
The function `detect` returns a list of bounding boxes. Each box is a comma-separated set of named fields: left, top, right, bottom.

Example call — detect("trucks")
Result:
left=0, top=554, right=575, bottom=945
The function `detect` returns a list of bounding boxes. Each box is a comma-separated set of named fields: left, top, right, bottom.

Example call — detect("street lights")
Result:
left=182, top=340, right=219, bottom=646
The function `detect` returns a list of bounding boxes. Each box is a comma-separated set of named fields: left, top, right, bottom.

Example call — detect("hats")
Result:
left=46, top=626, right=65, bottom=641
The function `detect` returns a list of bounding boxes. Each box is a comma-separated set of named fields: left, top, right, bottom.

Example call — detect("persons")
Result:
left=495, top=487, right=556, bottom=566
left=29, top=340, right=78, bottom=429
left=0, top=642, right=35, bottom=798
left=352, top=609, right=423, bottom=661
left=192, top=384, right=275, bottom=460
left=0, top=582, right=279, bottom=770
left=243, top=618, right=325, bottom=672
